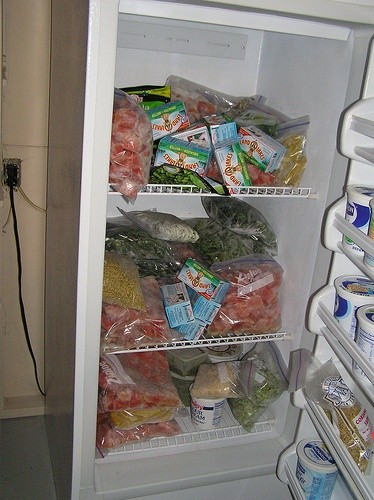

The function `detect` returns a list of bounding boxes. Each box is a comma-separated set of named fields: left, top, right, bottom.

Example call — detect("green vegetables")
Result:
left=148, top=164, right=210, bottom=195
left=105, top=196, right=277, bottom=284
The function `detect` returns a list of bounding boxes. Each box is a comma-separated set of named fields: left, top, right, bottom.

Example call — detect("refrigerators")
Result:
left=43, top=0, right=374, bottom=500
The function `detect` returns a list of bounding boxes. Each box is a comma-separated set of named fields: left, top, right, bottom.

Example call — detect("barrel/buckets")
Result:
left=350, top=301, right=374, bottom=381
left=189, top=382, right=227, bottom=432
left=296, top=437, right=341, bottom=500
left=342, top=183, right=374, bottom=257
left=331, top=273, right=373, bottom=339
left=358, top=197, right=374, bottom=272
